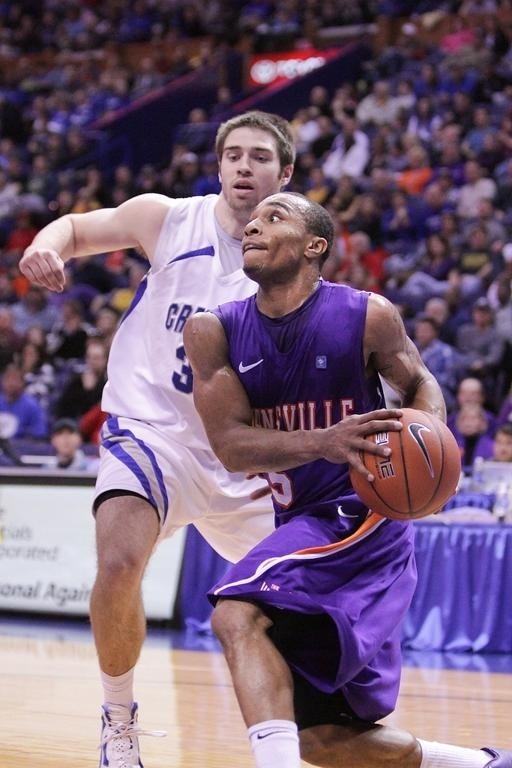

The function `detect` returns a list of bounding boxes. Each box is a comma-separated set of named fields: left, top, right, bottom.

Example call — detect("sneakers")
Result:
left=100, top=701, right=143, bottom=768
left=481, top=747, right=512, bottom=768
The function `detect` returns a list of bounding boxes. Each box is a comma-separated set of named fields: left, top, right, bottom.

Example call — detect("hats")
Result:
left=52, top=420, right=76, bottom=432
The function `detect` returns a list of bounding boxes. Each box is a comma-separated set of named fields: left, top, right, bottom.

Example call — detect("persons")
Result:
left=0, top=0, right=512, bottom=486
left=182, top=193, right=512, bottom=768
left=18, top=109, right=295, bottom=767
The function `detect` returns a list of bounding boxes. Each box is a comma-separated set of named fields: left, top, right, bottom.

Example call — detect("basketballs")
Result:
left=349, top=407, right=462, bottom=519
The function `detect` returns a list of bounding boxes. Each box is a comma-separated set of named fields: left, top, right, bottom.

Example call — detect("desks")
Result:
left=0, top=470, right=510, bottom=655
